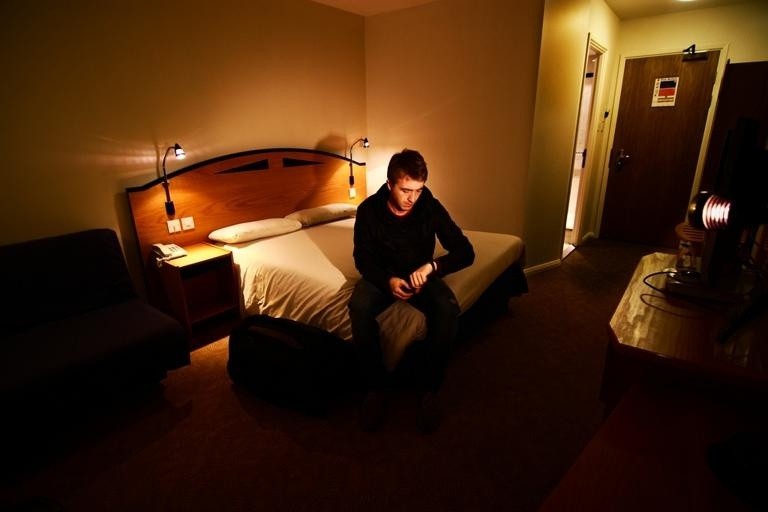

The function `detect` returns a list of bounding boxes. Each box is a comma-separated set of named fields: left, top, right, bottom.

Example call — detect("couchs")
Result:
left=0, top=229, right=190, bottom=481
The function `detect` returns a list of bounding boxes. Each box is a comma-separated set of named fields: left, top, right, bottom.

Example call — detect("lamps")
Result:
left=348, top=138, right=369, bottom=188
left=161, top=142, right=186, bottom=216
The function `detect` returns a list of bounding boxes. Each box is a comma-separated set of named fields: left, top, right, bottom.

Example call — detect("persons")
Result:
left=347, top=150, right=474, bottom=434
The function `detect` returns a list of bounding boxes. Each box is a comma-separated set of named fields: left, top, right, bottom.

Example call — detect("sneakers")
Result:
left=358, top=389, right=386, bottom=433
left=416, top=391, right=441, bottom=433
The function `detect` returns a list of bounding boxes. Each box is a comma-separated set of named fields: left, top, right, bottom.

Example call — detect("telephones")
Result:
left=150, top=243, right=187, bottom=260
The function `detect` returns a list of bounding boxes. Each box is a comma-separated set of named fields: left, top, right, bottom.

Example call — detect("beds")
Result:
left=124, top=148, right=529, bottom=397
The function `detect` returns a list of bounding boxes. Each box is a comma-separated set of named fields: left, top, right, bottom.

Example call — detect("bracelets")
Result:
left=428, top=260, right=435, bottom=273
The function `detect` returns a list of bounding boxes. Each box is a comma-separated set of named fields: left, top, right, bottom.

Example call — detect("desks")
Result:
left=593, top=252, right=768, bottom=399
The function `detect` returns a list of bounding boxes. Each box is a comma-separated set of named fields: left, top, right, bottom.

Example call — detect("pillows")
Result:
left=208, top=219, right=303, bottom=244
left=284, top=203, right=358, bottom=228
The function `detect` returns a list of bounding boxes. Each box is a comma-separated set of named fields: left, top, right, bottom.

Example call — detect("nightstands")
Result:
left=155, top=243, right=239, bottom=347
